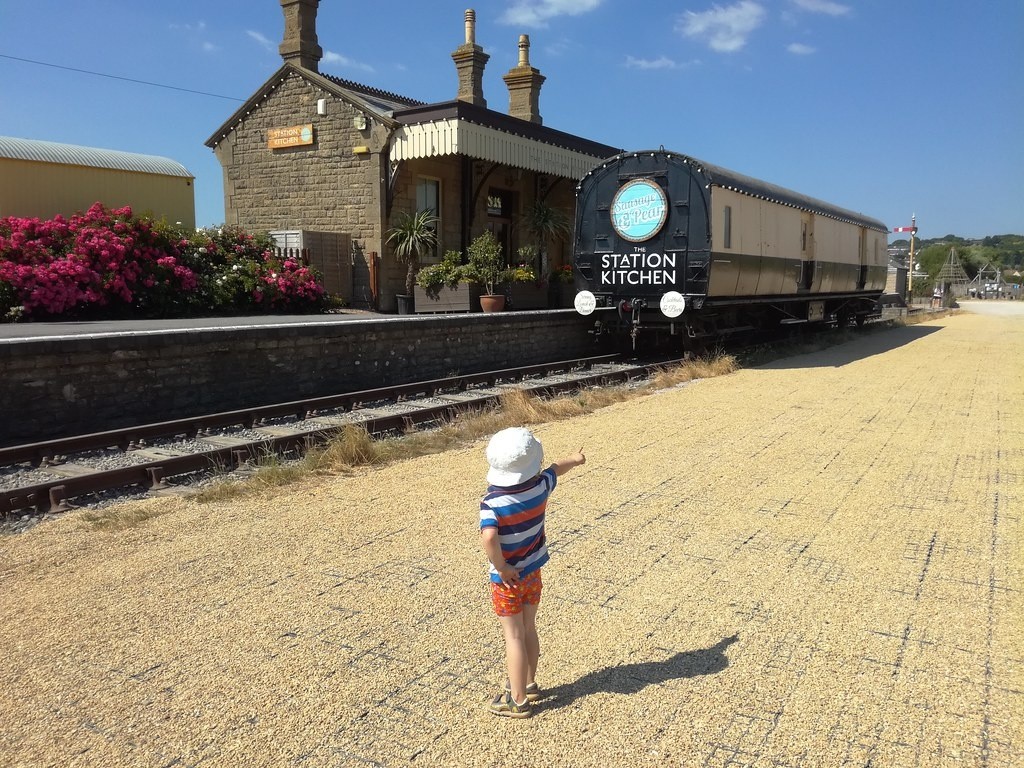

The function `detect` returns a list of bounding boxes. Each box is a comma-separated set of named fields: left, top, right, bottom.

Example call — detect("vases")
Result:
left=549, top=282, right=575, bottom=307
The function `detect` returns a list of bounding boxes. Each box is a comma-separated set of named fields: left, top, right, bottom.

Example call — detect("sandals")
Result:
left=487, top=691, right=533, bottom=718
left=506, top=680, right=540, bottom=702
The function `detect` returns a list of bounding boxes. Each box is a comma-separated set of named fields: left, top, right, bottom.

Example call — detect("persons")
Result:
left=478, top=426, right=590, bottom=714
left=933, top=286, right=984, bottom=307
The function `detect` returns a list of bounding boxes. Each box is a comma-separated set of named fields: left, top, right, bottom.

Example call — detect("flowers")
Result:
left=551, top=260, right=574, bottom=283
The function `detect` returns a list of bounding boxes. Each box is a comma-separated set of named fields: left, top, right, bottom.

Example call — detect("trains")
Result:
left=570, top=145, right=890, bottom=352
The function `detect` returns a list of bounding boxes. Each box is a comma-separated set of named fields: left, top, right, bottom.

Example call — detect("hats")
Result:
left=486, top=426, right=543, bottom=487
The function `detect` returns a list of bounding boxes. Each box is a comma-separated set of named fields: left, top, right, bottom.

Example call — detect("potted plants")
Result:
left=416, top=229, right=535, bottom=313
left=382, top=209, right=442, bottom=315
